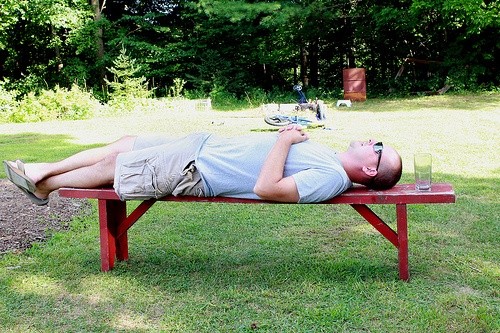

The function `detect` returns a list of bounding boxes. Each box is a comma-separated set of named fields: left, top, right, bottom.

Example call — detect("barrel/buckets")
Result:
left=343, top=68, right=366, bottom=102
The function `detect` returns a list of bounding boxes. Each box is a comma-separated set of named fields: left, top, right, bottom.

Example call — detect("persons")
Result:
left=1, top=123, right=402, bottom=206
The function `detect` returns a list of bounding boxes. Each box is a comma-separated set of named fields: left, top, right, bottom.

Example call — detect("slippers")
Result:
left=15, top=185, right=50, bottom=205
left=1, top=160, right=36, bottom=193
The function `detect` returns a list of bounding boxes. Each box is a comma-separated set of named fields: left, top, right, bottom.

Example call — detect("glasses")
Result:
left=368, top=141, right=382, bottom=183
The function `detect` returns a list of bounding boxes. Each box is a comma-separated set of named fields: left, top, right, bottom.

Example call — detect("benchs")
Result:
left=57, top=182, right=457, bottom=283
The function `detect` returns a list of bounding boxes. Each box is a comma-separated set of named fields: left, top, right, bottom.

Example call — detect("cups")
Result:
left=414, top=153, right=432, bottom=192
